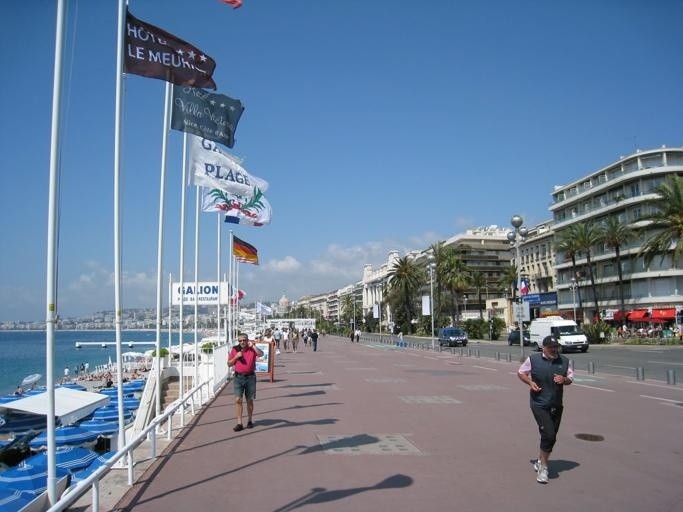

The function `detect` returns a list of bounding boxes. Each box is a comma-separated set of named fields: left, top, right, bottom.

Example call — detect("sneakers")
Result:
left=247, top=422, right=253, bottom=428
left=233, top=424, right=243, bottom=431
left=534, top=462, right=549, bottom=483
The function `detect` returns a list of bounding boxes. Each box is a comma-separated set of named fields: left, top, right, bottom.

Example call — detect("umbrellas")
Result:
left=21, top=373, right=42, bottom=386
left=0, top=378, right=146, bottom=512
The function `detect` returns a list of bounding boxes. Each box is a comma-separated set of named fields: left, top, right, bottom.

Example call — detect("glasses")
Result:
left=239, top=340, right=246, bottom=342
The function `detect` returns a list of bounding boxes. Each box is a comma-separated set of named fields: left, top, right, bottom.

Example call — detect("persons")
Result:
left=227, top=333, right=264, bottom=431
left=397, top=331, right=404, bottom=342
left=616, top=323, right=681, bottom=338
left=120, top=366, right=148, bottom=383
left=79, top=371, right=112, bottom=382
left=73, top=361, right=89, bottom=376
left=517, top=336, right=575, bottom=483
left=264, top=326, right=326, bottom=355
left=349, top=328, right=362, bottom=343
left=64, top=366, right=71, bottom=382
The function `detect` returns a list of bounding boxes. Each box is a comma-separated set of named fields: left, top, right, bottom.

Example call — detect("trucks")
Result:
left=530, top=315, right=588, bottom=352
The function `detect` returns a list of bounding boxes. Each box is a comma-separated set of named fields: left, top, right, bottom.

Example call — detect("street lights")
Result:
left=376, top=275, right=382, bottom=339
left=463, top=295, right=468, bottom=320
left=569, top=277, right=578, bottom=321
left=507, top=213, right=528, bottom=361
left=426, top=251, right=436, bottom=349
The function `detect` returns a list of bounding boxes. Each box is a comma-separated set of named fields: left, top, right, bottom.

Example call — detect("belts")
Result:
left=237, top=373, right=254, bottom=376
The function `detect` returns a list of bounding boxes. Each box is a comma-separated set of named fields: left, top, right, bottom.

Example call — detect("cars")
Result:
left=508, top=330, right=529, bottom=346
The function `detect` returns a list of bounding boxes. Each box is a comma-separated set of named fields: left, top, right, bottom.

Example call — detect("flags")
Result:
left=227, top=283, right=247, bottom=305
left=122, top=8, right=218, bottom=88
left=187, top=132, right=252, bottom=196
left=202, top=174, right=274, bottom=227
left=256, top=300, right=273, bottom=316
left=233, top=234, right=259, bottom=266
left=521, top=277, right=529, bottom=296
left=171, top=83, right=244, bottom=148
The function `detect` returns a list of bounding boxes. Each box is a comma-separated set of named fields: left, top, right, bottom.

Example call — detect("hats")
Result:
left=543, top=336, right=561, bottom=347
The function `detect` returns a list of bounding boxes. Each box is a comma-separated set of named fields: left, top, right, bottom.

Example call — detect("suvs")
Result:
left=437, top=327, right=467, bottom=346
